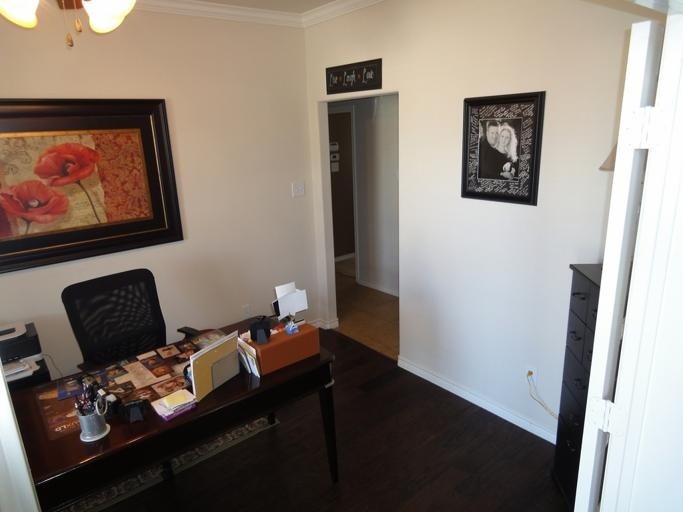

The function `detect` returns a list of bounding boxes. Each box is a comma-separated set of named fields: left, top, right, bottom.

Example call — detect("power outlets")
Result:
left=293, top=182, right=305, bottom=198
left=524, top=367, right=538, bottom=386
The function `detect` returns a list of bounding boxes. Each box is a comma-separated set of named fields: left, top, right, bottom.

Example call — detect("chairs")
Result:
left=62, top=269, right=167, bottom=372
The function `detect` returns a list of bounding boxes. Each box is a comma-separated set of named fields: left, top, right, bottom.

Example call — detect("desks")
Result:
left=10, top=316, right=339, bottom=512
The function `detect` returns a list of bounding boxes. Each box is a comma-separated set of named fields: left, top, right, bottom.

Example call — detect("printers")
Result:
left=1, top=320, right=44, bottom=383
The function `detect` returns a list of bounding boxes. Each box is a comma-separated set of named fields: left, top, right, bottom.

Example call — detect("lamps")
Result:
left=0, top=0, right=136, bottom=45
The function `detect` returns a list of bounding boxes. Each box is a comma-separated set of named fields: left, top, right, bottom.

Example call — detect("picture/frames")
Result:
left=0, top=98, right=184, bottom=274
left=461, top=91, right=545, bottom=206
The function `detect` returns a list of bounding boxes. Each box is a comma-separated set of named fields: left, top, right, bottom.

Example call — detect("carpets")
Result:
left=58, top=413, right=332, bottom=511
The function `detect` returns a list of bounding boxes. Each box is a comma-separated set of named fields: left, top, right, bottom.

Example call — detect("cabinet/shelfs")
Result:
left=554, top=262, right=603, bottom=504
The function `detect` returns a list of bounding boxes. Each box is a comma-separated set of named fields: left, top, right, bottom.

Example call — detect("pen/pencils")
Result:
left=74, top=394, right=98, bottom=416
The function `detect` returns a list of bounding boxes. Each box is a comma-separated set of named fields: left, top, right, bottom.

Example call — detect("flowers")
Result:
left=34, top=144, right=103, bottom=223
left=1, top=180, right=69, bottom=235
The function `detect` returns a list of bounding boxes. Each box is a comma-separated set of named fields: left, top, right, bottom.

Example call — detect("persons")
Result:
left=477, top=121, right=518, bottom=181
